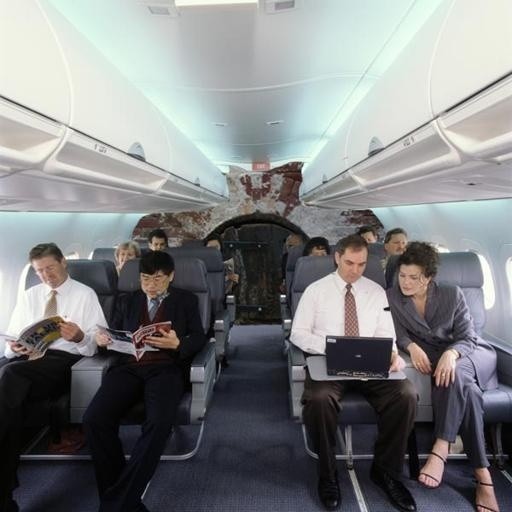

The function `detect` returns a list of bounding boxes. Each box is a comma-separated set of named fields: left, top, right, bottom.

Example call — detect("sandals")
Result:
left=473, top=480, right=500, bottom=512
left=418, top=450, right=447, bottom=489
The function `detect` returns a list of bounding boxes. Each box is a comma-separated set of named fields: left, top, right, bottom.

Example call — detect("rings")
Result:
left=417, top=363, right=420, bottom=366
left=441, top=373, right=445, bottom=377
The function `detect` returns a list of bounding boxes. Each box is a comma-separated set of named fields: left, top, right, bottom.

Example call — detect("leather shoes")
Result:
left=318, top=467, right=340, bottom=508
left=370, top=462, right=416, bottom=512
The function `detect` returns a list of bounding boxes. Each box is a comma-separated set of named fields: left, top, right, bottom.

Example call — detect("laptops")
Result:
left=325, top=334, right=393, bottom=379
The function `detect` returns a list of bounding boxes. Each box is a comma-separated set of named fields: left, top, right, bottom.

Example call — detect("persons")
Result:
left=1, top=242, right=109, bottom=512
left=381, top=228, right=409, bottom=273
left=83, top=252, right=204, bottom=512
left=302, top=237, right=329, bottom=256
left=389, top=242, right=512, bottom=511
left=291, top=236, right=419, bottom=511
left=279, top=235, right=301, bottom=294
left=358, top=226, right=378, bottom=243
left=113, top=240, right=140, bottom=277
left=146, top=229, right=168, bottom=250
left=205, top=236, right=241, bottom=287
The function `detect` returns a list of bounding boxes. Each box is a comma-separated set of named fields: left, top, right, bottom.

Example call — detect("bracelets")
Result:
left=449, top=349, right=458, bottom=358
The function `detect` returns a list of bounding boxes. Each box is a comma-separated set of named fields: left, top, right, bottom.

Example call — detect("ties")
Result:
left=147, top=300, right=160, bottom=322
left=345, top=284, right=359, bottom=337
left=44, top=290, right=56, bottom=319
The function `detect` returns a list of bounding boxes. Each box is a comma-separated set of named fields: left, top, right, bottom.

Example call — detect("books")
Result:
left=223, top=258, right=234, bottom=295
left=0, top=316, right=66, bottom=359
left=98, top=322, right=171, bottom=362
